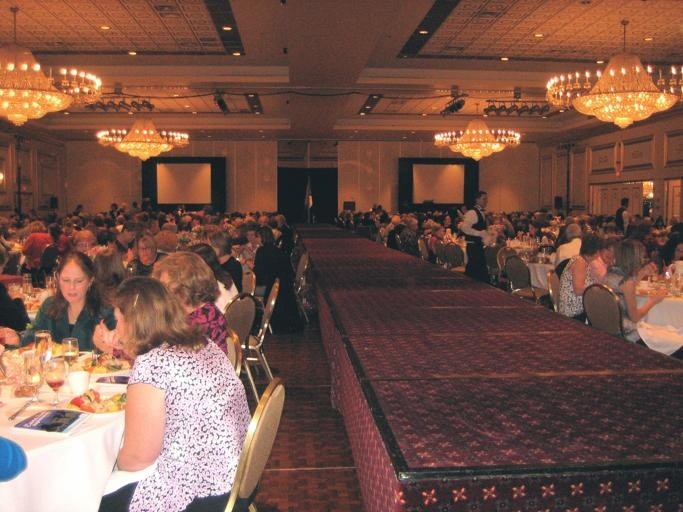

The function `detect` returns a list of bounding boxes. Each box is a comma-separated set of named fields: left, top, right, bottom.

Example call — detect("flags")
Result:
left=304, top=181, right=314, bottom=224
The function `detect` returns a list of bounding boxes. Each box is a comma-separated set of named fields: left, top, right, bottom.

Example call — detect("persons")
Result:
left=339, top=190, right=683, bottom=345
left=99, top=276, right=253, bottom=511
left=0, top=198, right=292, bottom=359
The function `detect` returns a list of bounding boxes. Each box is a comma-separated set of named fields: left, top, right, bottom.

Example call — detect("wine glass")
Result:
left=18, top=329, right=78, bottom=408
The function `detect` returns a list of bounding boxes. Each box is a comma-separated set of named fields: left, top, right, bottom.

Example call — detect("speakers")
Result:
left=50, top=197, right=57, bottom=209
left=555, top=196, right=562, bottom=208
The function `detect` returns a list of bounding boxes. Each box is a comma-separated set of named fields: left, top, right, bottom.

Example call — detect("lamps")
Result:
left=210, top=94, right=230, bottom=117
left=484, top=100, right=550, bottom=116
left=543, top=17, right=682, bottom=131
left=83, top=96, right=155, bottom=113
left=432, top=98, right=525, bottom=165
left=439, top=95, right=465, bottom=118
left=95, top=117, right=189, bottom=165
left=0, top=6, right=110, bottom=129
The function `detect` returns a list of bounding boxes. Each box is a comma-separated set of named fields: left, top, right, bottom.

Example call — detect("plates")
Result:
left=78, top=391, right=123, bottom=414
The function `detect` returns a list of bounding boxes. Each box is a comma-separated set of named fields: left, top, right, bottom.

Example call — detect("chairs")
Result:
left=290, top=237, right=308, bottom=320
left=581, top=283, right=640, bottom=340
left=223, top=256, right=279, bottom=406
left=358, top=223, right=466, bottom=273
left=485, top=238, right=563, bottom=311
left=222, top=375, right=287, bottom=512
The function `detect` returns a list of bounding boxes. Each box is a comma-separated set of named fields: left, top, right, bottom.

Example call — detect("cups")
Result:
left=127, top=267, right=135, bottom=279
left=646, top=271, right=681, bottom=297
left=505, top=234, right=549, bottom=261
left=6, top=272, right=56, bottom=292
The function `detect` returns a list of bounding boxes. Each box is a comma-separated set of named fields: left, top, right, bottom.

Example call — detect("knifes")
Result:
left=8, top=400, right=34, bottom=421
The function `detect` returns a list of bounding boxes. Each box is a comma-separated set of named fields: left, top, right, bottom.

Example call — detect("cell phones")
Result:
left=96, top=375, right=129, bottom=384
